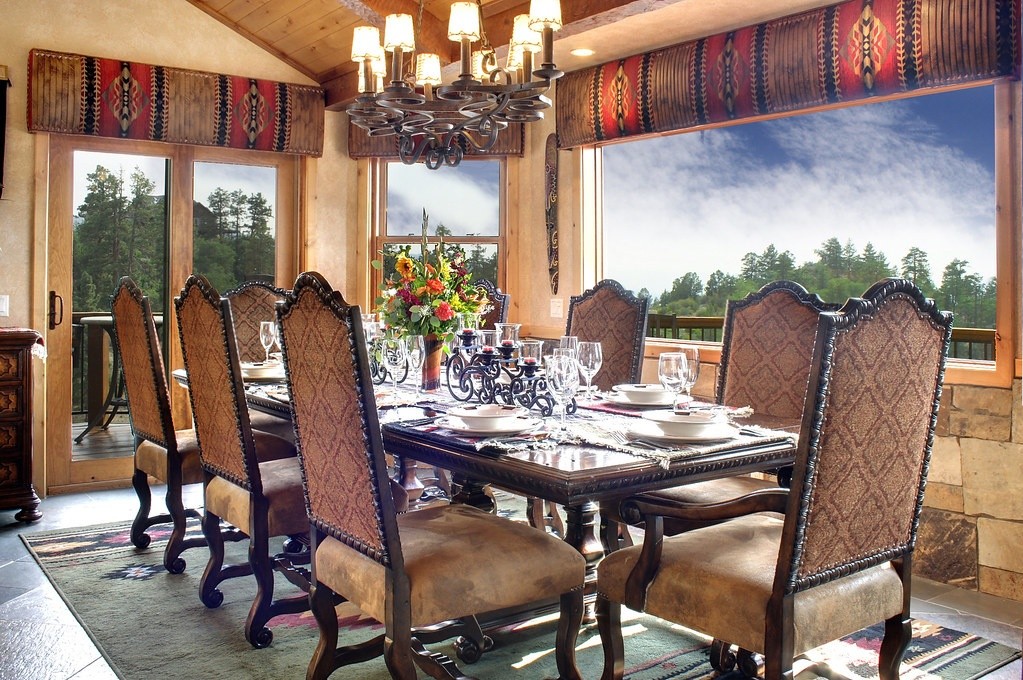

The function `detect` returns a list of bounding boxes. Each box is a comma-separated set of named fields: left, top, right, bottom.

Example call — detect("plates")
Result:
left=242, top=374, right=285, bottom=380
left=606, top=393, right=695, bottom=409
left=434, top=415, right=544, bottom=439
left=629, top=429, right=738, bottom=439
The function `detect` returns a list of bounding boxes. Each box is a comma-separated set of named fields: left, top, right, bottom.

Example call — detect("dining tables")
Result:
left=176, top=356, right=802, bottom=680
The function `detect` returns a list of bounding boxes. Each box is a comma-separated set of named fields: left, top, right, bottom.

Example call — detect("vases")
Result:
left=422, top=333, right=445, bottom=391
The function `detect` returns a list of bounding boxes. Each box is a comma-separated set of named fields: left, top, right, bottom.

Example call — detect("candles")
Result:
left=502, top=340, right=512, bottom=345
left=462, top=329, right=473, bottom=334
left=525, top=355, right=537, bottom=362
left=483, top=347, right=492, bottom=351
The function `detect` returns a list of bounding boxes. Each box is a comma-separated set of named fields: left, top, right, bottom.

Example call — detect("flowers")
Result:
left=374, top=207, right=494, bottom=354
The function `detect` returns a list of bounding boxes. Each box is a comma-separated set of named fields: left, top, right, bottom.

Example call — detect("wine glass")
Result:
left=658, top=347, right=700, bottom=412
left=259, top=320, right=282, bottom=365
left=476, top=323, right=603, bottom=442
left=381, top=335, right=426, bottom=417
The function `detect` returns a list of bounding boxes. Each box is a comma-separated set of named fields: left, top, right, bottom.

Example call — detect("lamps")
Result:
left=348, top=0, right=563, bottom=168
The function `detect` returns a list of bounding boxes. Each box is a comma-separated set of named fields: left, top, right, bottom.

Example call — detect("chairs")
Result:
left=645, top=312, right=677, bottom=338
left=108, top=269, right=955, bottom=679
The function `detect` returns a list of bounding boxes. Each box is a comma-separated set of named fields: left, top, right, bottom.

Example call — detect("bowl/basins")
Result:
left=643, top=412, right=715, bottom=437
left=241, top=360, right=277, bottom=377
left=447, top=403, right=532, bottom=430
left=611, top=383, right=670, bottom=404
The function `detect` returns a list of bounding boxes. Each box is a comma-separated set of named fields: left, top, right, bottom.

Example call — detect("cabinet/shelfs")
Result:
left=0, top=329, right=43, bottom=522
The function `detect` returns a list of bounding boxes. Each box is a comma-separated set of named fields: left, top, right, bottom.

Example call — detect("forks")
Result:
left=612, top=431, right=671, bottom=450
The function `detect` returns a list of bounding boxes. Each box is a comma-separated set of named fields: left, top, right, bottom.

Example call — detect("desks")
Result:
left=76, top=314, right=166, bottom=444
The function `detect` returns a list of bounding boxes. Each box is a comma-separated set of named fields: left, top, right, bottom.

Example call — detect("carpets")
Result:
left=23, top=489, right=1023, bottom=680
